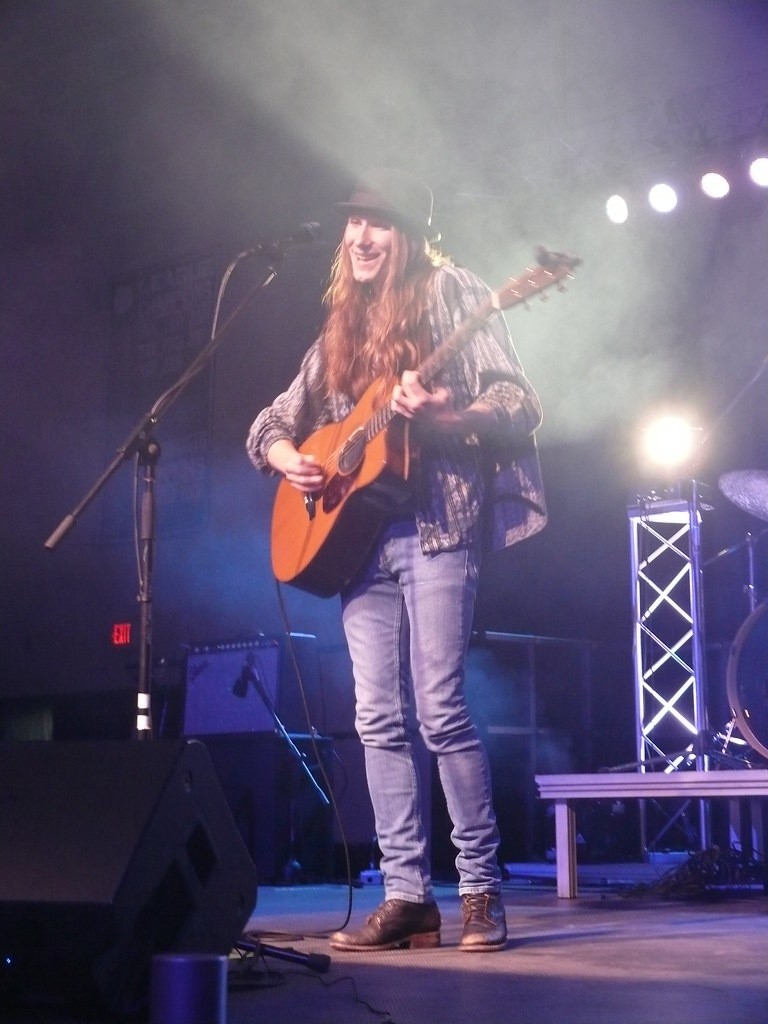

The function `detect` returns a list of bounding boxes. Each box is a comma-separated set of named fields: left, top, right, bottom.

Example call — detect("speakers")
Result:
left=201, top=732, right=331, bottom=886
left=0, top=736, right=256, bottom=1003
left=183, top=633, right=320, bottom=738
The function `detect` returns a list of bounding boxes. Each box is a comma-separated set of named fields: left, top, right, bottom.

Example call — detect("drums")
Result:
left=726, top=596, right=768, bottom=760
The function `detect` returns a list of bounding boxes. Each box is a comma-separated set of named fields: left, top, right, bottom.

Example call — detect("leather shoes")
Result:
left=326, top=899, right=443, bottom=950
left=458, top=894, right=509, bottom=952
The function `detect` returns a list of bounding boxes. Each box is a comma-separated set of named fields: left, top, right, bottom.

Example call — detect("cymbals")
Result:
left=718, top=467, right=767, bottom=522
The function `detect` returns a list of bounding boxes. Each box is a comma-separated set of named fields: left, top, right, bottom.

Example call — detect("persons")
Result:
left=244, top=171, right=545, bottom=951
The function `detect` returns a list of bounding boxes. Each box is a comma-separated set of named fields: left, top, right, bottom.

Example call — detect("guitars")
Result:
left=268, top=243, right=588, bottom=600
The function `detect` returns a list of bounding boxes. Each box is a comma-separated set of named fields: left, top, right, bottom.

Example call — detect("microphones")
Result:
left=232, top=652, right=253, bottom=698
left=236, top=223, right=322, bottom=264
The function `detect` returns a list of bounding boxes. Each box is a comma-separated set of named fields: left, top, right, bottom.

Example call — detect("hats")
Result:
left=332, top=167, right=442, bottom=244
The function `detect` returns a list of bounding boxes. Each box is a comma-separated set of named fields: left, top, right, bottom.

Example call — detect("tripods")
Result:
left=596, top=354, right=768, bottom=774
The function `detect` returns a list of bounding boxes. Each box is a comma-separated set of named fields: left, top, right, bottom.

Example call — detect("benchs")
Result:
left=534, top=769, right=768, bottom=898
